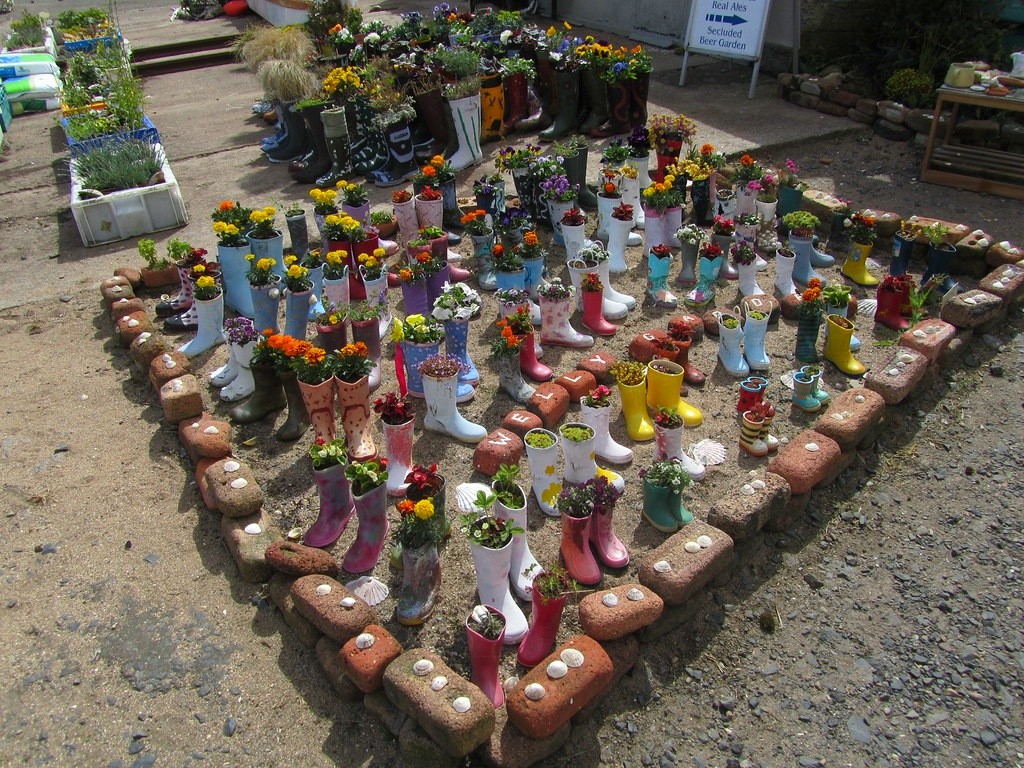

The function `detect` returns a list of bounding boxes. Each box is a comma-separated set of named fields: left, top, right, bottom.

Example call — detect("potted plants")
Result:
left=1, top=8, right=192, bottom=249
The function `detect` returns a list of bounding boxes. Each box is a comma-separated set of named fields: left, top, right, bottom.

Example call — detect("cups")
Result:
left=944, top=62, right=975, bottom=88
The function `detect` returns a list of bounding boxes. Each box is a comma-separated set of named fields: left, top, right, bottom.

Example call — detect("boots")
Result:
left=517, top=574, right=566, bottom=667
left=642, top=463, right=693, bottom=533
left=652, top=415, right=706, bottom=482
left=524, top=422, right=625, bottom=517
left=740, top=403, right=780, bottom=455
left=840, top=234, right=880, bottom=287
left=561, top=481, right=629, bottom=587
left=887, top=229, right=918, bottom=282
left=252, top=7, right=649, bottom=188
left=470, top=480, right=545, bottom=644
left=156, top=174, right=487, bottom=626
left=652, top=331, right=705, bottom=397
left=618, top=360, right=703, bottom=441
left=920, top=239, right=963, bottom=294
left=875, top=280, right=930, bottom=331
left=823, top=295, right=866, bottom=378
left=579, top=396, right=633, bottom=464
left=792, top=366, right=830, bottom=412
left=737, top=377, right=775, bottom=416
left=466, top=604, right=507, bottom=707
left=469, top=131, right=834, bottom=403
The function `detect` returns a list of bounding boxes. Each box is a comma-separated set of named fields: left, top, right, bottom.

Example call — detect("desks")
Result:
left=918, top=74, right=1024, bottom=200
